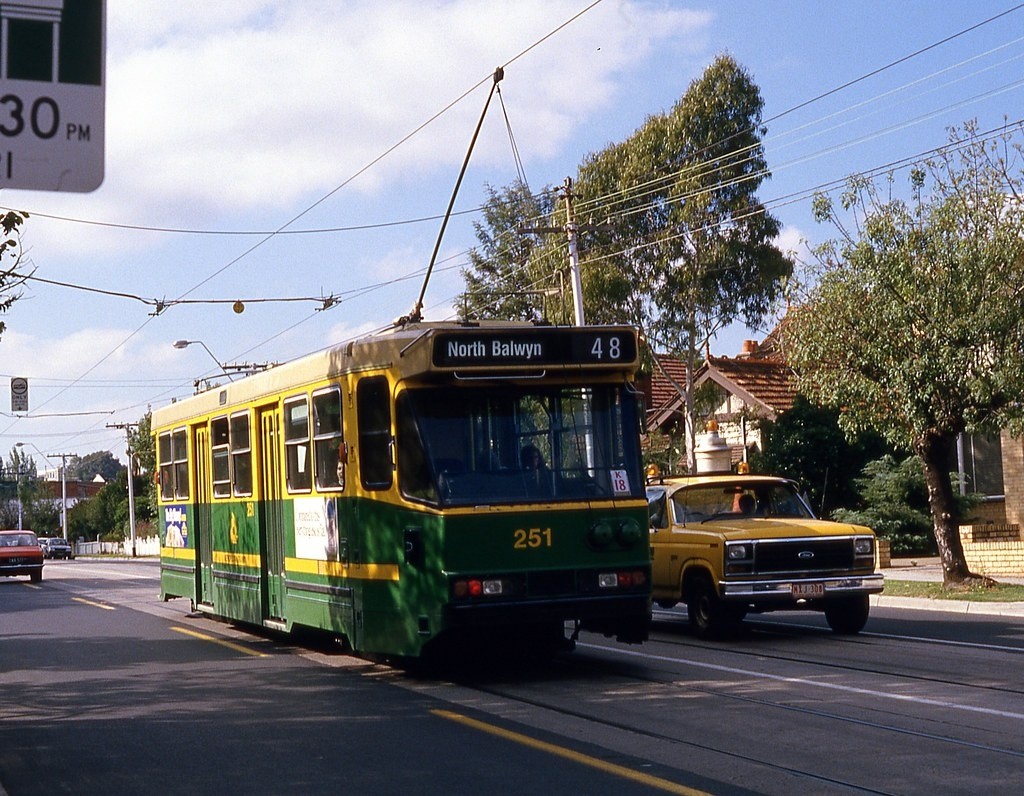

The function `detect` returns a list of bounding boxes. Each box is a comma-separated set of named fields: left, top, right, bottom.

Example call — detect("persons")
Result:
left=520, top=445, right=539, bottom=470
left=328, top=459, right=343, bottom=487
left=739, top=494, right=755, bottom=514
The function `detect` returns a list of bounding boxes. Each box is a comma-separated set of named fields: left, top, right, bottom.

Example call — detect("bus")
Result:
left=151, top=322, right=652, bottom=660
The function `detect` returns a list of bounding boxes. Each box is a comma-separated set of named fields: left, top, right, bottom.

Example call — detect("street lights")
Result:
left=171, top=340, right=234, bottom=384
left=16, top=442, right=66, bottom=542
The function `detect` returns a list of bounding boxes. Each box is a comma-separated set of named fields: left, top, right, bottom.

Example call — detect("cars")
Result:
left=38, top=536, right=71, bottom=558
left=645, top=472, right=884, bottom=641
left=0, top=530, right=45, bottom=583
